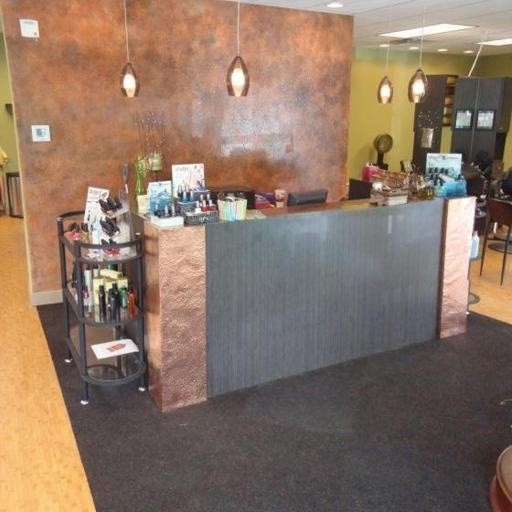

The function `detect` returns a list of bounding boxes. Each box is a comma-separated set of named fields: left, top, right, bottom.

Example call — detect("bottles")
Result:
left=72, top=222, right=89, bottom=242
left=470, top=231, right=480, bottom=258
left=149, top=179, right=215, bottom=220
left=133, top=166, right=147, bottom=212
left=81, top=262, right=134, bottom=322
left=430, top=166, right=465, bottom=187
left=487, top=219, right=497, bottom=239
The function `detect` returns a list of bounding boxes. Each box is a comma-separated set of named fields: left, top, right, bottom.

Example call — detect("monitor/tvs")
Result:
left=287, top=190, right=328, bottom=206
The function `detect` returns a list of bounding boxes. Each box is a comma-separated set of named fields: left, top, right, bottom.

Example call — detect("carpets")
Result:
left=35, top=303, right=512, bottom=512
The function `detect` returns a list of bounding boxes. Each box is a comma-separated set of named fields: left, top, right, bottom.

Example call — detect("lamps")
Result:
left=377, top=0, right=431, bottom=106
left=120, top=0, right=140, bottom=98
left=226, top=0, right=250, bottom=98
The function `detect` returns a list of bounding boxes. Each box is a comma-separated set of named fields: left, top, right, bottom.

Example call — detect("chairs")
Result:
left=400, top=158, right=512, bottom=288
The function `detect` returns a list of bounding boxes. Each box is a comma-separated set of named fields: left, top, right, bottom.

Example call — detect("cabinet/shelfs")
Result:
left=411, top=74, right=459, bottom=174
left=449, top=77, right=512, bottom=173
left=54, top=208, right=152, bottom=406
